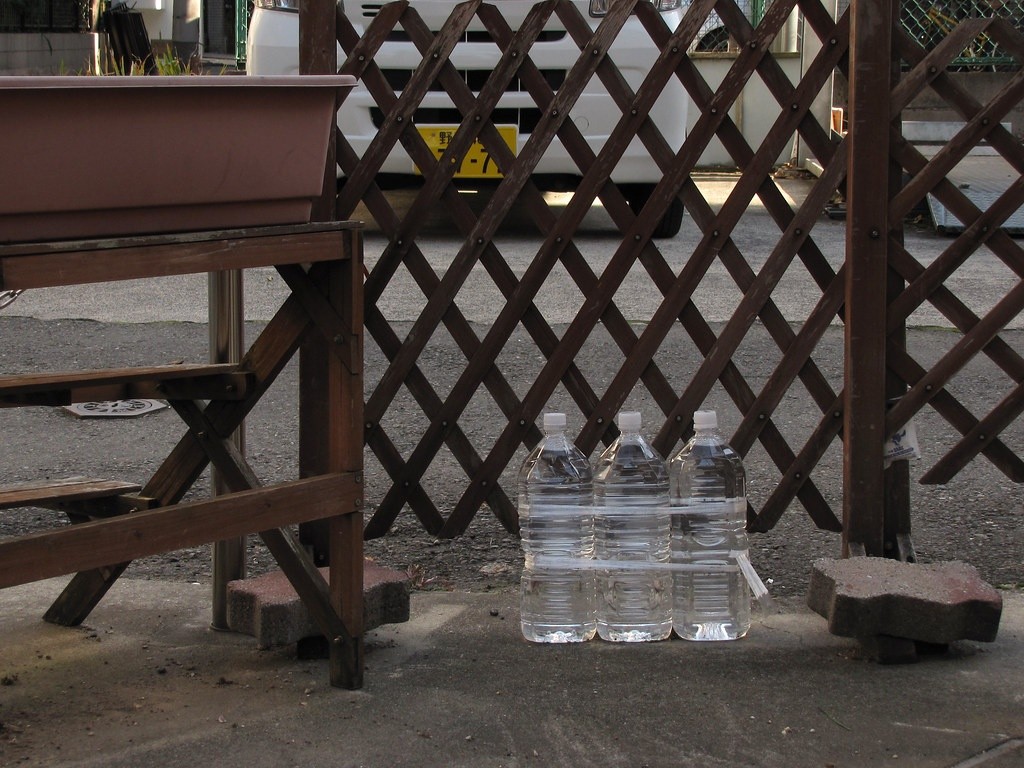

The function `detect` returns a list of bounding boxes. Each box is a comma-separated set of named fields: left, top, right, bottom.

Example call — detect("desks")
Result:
left=0, top=218, right=366, bottom=696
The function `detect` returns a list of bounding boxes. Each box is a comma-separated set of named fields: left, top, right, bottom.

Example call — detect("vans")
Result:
left=244, top=1, right=693, bottom=239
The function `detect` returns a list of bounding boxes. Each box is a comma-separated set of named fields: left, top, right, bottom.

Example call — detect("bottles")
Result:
left=593, top=412, right=673, bottom=643
left=515, top=412, right=595, bottom=645
left=670, top=410, right=751, bottom=642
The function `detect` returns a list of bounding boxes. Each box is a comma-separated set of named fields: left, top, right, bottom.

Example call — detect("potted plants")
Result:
left=0, top=52, right=358, bottom=241
